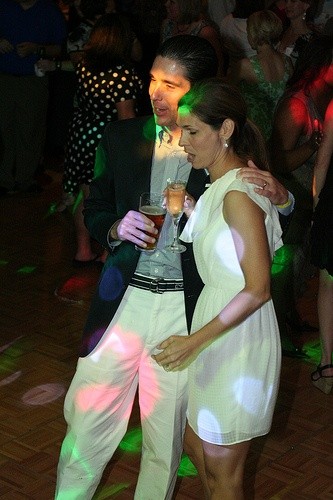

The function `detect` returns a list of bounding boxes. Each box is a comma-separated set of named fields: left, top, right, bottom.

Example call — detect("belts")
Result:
left=129, top=274, right=184, bottom=295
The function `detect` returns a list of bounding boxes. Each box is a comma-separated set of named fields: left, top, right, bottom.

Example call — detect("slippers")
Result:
left=73, top=252, right=102, bottom=267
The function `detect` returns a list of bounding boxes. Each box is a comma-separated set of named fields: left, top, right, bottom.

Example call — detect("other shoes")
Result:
left=24, top=185, right=45, bottom=194
left=289, top=350, right=310, bottom=358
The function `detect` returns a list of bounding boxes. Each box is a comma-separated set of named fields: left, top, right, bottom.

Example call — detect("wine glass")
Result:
left=163, top=176, right=186, bottom=254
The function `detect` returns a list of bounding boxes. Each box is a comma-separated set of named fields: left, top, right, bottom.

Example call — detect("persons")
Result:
left=0, top=0, right=333, bottom=394
left=54, top=35, right=312, bottom=500
left=152, top=78, right=283, bottom=499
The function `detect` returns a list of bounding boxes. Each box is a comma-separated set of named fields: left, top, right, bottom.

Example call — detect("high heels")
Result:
left=311, top=363, right=333, bottom=392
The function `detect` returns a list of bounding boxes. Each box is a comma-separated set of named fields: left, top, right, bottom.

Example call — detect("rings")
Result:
left=261, top=181, right=268, bottom=189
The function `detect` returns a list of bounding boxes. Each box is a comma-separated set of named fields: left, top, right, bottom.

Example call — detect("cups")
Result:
left=135, top=193, right=167, bottom=253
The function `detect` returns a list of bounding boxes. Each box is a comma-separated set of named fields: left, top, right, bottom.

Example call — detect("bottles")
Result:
left=35, top=47, right=45, bottom=77
